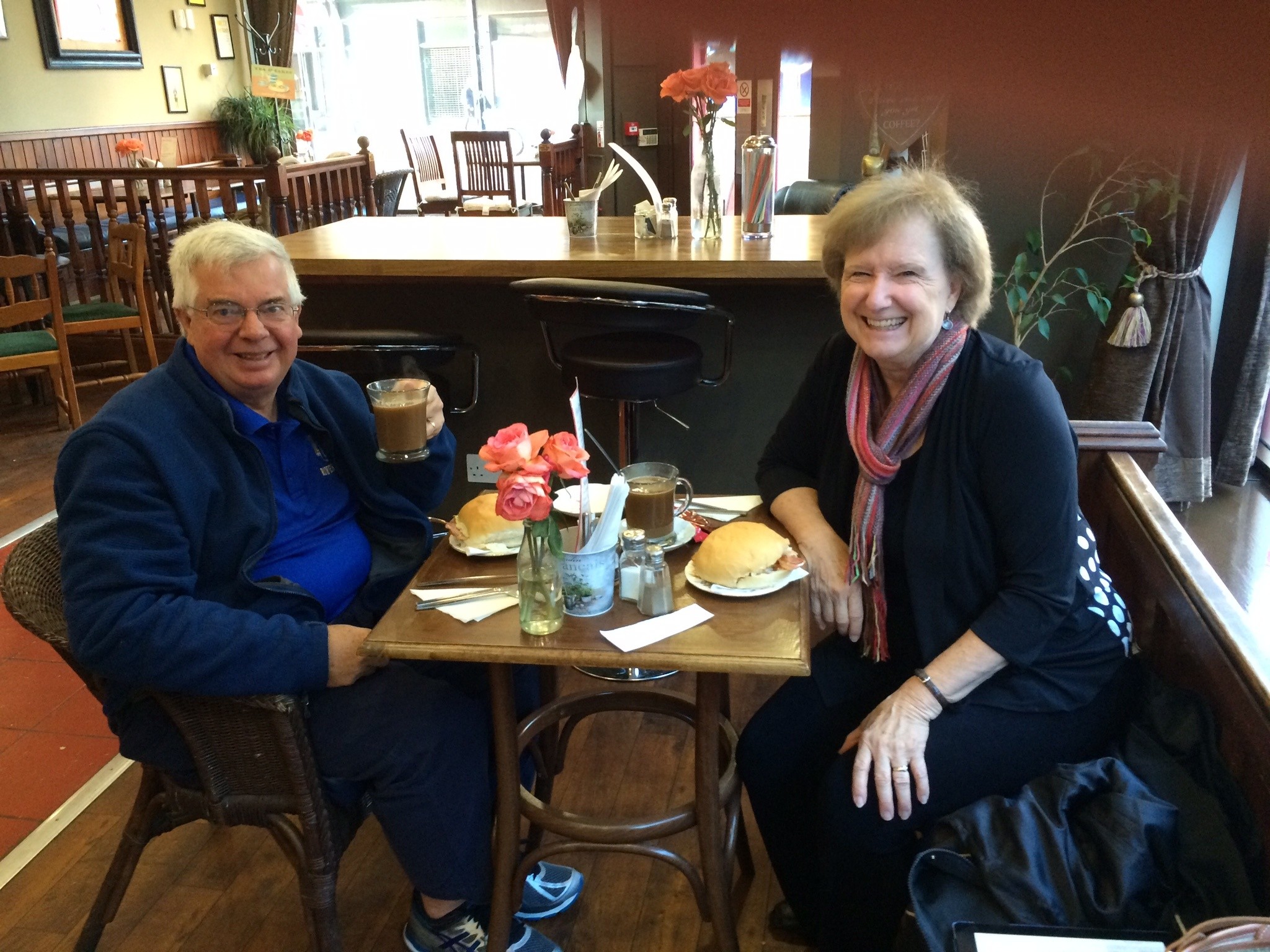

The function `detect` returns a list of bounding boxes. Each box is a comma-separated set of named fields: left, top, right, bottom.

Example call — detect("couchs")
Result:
left=773, top=180, right=856, bottom=216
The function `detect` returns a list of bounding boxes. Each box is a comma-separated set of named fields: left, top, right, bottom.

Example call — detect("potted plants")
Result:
left=212, top=85, right=300, bottom=164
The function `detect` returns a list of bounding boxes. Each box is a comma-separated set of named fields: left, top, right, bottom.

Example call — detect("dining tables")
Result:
left=464, top=156, right=542, bottom=202
left=357, top=491, right=812, bottom=952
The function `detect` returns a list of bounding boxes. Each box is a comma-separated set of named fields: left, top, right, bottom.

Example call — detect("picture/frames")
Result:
left=32, top=0, right=235, bottom=114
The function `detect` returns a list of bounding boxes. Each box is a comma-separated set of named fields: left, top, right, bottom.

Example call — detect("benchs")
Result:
left=1070, top=415, right=1269, bottom=923
left=30, top=192, right=265, bottom=305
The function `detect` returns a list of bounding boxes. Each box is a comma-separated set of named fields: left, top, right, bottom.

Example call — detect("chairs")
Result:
left=372, top=166, right=414, bottom=216
left=0, top=519, right=373, bottom=951
left=451, top=129, right=533, bottom=217
left=399, top=126, right=482, bottom=217
left=1, top=237, right=81, bottom=430
left=43, top=209, right=159, bottom=431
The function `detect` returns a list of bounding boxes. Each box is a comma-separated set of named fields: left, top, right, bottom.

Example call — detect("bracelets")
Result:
left=913, top=668, right=951, bottom=709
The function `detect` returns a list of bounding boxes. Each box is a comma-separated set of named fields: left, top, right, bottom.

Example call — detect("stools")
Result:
left=511, top=278, right=734, bottom=469
left=292, top=326, right=480, bottom=418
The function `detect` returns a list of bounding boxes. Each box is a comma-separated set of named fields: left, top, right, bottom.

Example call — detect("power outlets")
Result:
left=467, top=453, right=503, bottom=484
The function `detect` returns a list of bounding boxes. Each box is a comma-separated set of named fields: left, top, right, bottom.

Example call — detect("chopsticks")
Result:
left=673, top=497, right=747, bottom=516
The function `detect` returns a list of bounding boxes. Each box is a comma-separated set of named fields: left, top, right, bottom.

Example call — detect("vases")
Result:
left=516, top=521, right=565, bottom=635
left=303, top=143, right=314, bottom=162
left=691, top=137, right=723, bottom=239
left=128, top=160, right=145, bottom=192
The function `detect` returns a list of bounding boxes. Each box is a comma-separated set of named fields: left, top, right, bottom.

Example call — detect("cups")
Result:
left=552, top=525, right=616, bottom=617
left=564, top=195, right=599, bottom=239
left=367, top=377, right=435, bottom=461
left=634, top=210, right=658, bottom=240
left=617, top=462, right=693, bottom=544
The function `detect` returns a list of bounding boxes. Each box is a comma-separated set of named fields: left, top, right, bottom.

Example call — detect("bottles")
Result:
left=657, top=198, right=678, bottom=238
left=515, top=519, right=565, bottom=635
left=615, top=530, right=674, bottom=616
left=130, top=162, right=145, bottom=190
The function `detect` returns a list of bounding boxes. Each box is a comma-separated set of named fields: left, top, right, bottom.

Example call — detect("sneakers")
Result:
left=513, top=860, right=584, bottom=922
left=403, top=883, right=564, bottom=952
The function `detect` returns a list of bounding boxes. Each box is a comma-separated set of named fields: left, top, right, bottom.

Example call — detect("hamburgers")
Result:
left=693, top=519, right=807, bottom=589
left=445, top=492, right=525, bottom=547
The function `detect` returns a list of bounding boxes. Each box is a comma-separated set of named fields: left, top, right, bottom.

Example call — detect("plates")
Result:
left=447, top=532, right=525, bottom=559
left=618, top=517, right=696, bottom=556
left=684, top=555, right=793, bottom=598
left=553, top=483, right=614, bottom=517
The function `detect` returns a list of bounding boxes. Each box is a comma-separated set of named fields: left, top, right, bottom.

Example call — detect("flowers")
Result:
left=295, top=129, right=313, bottom=162
left=115, top=137, right=146, bottom=187
left=659, top=62, right=738, bottom=236
left=479, top=423, right=590, bottom=626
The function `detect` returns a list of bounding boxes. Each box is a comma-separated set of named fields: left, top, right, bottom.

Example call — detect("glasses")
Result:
left=188, top=300, right=300, bottom=326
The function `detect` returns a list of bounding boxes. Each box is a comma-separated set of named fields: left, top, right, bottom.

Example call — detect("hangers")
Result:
left=235, top=9, right=292, bottom=157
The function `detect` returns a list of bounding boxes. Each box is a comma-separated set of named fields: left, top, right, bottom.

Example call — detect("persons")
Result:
left=54, top=219, right=580, bottom=952
left=738, top=168, right=1141, bottom=951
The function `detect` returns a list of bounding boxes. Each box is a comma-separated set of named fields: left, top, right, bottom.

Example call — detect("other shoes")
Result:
left=770, top=898, right=809, bottom=938
